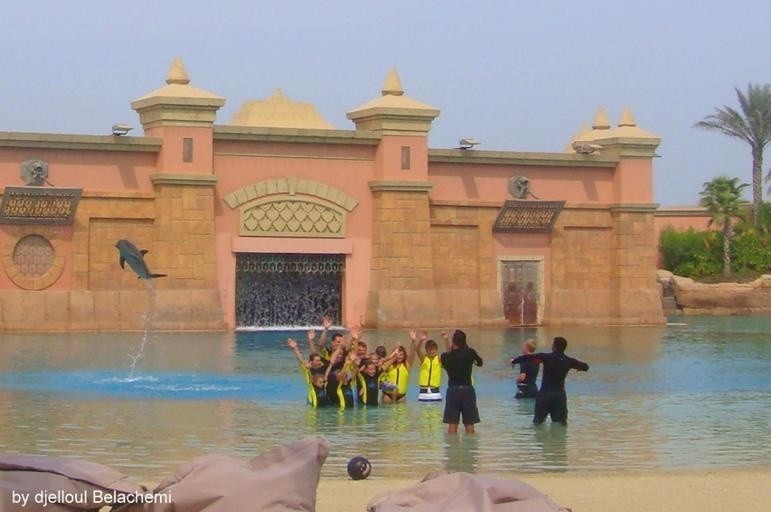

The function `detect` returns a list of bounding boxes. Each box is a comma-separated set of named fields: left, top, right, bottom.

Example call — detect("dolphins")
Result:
left=115, top=239, right=168, bottom=280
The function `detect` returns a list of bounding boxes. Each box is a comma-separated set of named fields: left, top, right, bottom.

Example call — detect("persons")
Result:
left=287, top=314, right=450, bottom=414
left=514, top=338, right=541, bottom=399
left=439, top=330, right=483, bottom=435
left=508, top=336, right=590, bottom=426
